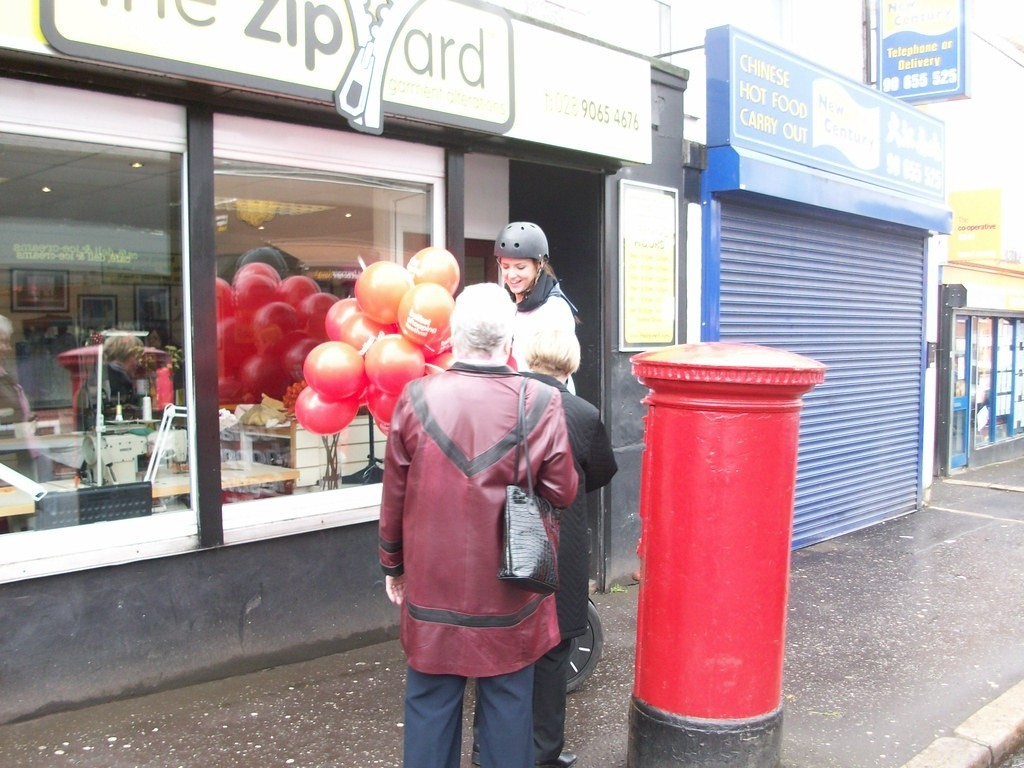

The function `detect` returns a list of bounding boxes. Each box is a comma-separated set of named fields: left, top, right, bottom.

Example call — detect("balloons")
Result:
left=294, top=246, right=461, bottom=433
left=215, top=247, right=343, bottom=409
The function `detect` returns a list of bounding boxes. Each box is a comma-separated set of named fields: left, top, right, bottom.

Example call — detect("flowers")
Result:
left=278, top=379, right=306, bottom=413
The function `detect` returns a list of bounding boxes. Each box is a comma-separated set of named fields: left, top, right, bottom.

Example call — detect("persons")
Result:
left=469, top=325, right=619, bottom=768
left=377, top=282, right=580, bottom=768
left=144, top=326, right=184, bottom=390
left=0, top=315, right=40, bottom=488
left=493, top=220, right=577, bottom=399
left=76, top=335, right=149, bottom=478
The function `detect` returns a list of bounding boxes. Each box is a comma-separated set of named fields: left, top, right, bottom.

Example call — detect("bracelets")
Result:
left=13, top=411, right=39, bottom=430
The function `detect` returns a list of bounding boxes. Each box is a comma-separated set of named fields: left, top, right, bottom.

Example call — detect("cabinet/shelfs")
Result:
left=221, top=406, right=387, bottom=488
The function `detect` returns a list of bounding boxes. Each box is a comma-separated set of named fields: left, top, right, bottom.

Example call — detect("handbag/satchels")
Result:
left=497, top=376, right=559, bottom=596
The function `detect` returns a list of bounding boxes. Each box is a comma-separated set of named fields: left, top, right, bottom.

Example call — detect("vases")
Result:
left=318, top=431, right=343, bottom=491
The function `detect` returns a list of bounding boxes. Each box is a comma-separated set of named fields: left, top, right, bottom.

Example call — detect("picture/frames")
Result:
left=77, top=293, right=118, bottom=331
left=10, top=268, right=70, bottom=312
left=133, top=282, right=173, bottom=339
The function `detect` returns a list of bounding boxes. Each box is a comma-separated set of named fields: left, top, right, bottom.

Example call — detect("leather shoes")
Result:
left=471, top=743, right=481, bottom=765
left=535, top=754, right=577, bottom=768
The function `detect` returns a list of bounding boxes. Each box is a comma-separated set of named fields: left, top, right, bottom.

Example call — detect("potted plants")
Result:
left=136, top=330, right=161, bottom=419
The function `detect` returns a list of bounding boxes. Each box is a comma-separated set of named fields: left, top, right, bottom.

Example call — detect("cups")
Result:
left=142, top=396, right=152, bottom=420
left=14, top=421, right=36, bottom=438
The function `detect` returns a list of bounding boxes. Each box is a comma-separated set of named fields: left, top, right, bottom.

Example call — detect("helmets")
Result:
left=494, top=222, right=550, bottom=261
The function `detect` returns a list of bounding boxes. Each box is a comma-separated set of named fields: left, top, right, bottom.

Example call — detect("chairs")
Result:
left=34, top=481, right=151, bottom=530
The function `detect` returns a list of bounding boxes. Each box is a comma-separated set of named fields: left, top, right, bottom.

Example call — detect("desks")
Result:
left=0, top=434, right=84, bottom=482
left=0, top=462, right=300, bottom=518
